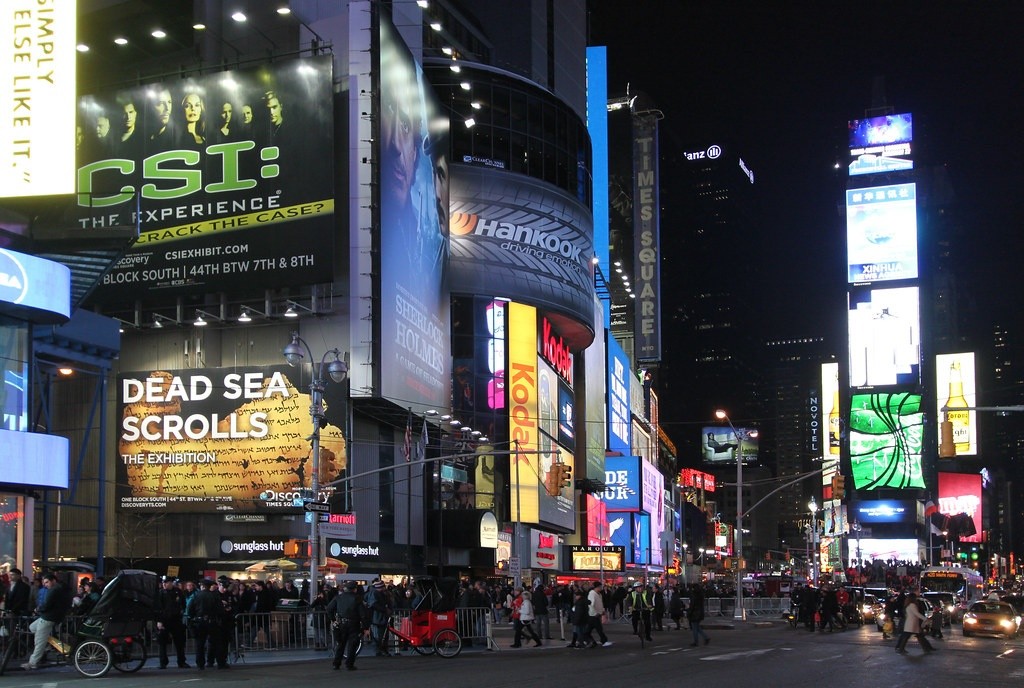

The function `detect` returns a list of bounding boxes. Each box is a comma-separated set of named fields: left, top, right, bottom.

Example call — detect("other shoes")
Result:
left=703, top=637, right=711, bottom=646
left=375, top=652, right=392, bottom=657
left=566, top=642, right=616, bottom=649
left=157, top=661, right=231, bottom=670
left=804, top=625, right=849, bottom=634
left=924, top=648, right=939, bottom=656
left=20, top=663, right=38, bottom=670
left=332, top=665, right=341, bottom=670
left=894, top=646, right=911, bottom=655
left=645, top=636, right=653, bottom=641
left=690, top=642, right=699, bottom=647
left=510, top=636, right=554, bottom=647
left=345, top=666, right=357, bottom=671
left=633, top=629, right=638, bottom=635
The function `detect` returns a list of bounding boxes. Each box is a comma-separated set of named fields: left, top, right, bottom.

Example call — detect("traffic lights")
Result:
left=833, top=474, right=847, bottom=499
left=558, top=462, right=572, bottom=488
left=972, top=552, right=979, bottom=568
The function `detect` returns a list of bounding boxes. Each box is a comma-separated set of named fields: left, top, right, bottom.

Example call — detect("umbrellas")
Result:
left=245, top=559, right=300, bottom=581
left=303, top=558, right=348, bottom=576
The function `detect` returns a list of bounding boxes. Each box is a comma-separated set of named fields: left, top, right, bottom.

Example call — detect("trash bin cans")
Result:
left=270, top=599, right=311, bottom=643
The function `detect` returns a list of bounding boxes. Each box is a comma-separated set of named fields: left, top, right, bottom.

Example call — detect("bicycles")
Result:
left=0, top=571, right=158, bottom=678
left=337, top=607, right=462, bottom=660
left=833, top=586, right=864, bottom=629
left=790, top=601, right=803, bottom=629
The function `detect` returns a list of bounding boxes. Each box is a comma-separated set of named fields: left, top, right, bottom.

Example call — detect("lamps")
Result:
left=407, top=45, right=456, bottom=55
left=447, top=104, right=478, bottom=131
left=75, top=41, right=133, bottom=73
left=149, top=25, right=200, bottom=62
left=445, top=426, right=473, bottom=432
left=151, top=313, right=177, bottom=330
left=453, top=430, right=482, bottom=437
left=393, top=0, right=429, bottom=8
left=193, top=308, right=220, bottom=327
left=231, top=11, right=281, bottom=54
left=432, top=420, right=462, bottom=426
left=440, top=97, right=484, bottom=112
left=114, top=316, right=135, bottom=333
left=611, top=261, right=635, bottom=300
left=471, top=437, right=491, bottom=444
left=284, top=300, right=312, bottom=318
left=427, top=415, right=451, bottom=422
left=420, top=62, right=462, bottom=73
left=394, top=18, right=451, bottom=33
left=276, top=3, right=328, bottom=49
left=433, top=80, right=472, bottom=91
left=109, top=33, right=161, bottom=66
left=191, top=20, right=237, bottom=55
left=415, top=409, right=439, bottom=417
left=238, top=304, right=264, bottom=323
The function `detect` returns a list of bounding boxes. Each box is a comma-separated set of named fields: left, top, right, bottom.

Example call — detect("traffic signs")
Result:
left=305, top=512, right=330, bottom=523
left=303, top=501, right=331, bottom=513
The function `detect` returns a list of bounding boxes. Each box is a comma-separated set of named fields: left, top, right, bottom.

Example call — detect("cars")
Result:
left=962, top=599, right=1022, bottom=639
left=863, top=594, right=884, bottom=623
left=877, top=598, right=934, bottom=635
left=928, top=599, right=952, bottom=627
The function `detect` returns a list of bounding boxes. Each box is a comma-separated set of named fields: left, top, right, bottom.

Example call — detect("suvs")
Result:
left=922, top=591, right=962, bottom=624
left=986, top=591, right=1015, bottom=605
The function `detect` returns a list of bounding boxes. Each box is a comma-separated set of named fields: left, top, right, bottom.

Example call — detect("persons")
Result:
left=457, top=576, right=552, bottom=649
left=326, top=582, right=370, bottom=670
left=627, top=582, right=653, bottom=643
left=300, top=578, right=418, bottom=656
left=153, top=573, right=299, bottom=671
left=852, top=574, right=867, bottom=588
left=0, top=563, right=114, bottom=672
left=1001, top=593, right=1024, bottom=615
left=650, top=580, right=736, bottom=633
left=686, top=583, right=710, bottom=647
left=554, top=578, right=631, bottom=649
left=792, top=581, right=849, bottom=633
left=75, top=81, right=289, bottom=185
left=881, top=592, right=943, bottom=656
left=708, top=432, right=738, bottom=453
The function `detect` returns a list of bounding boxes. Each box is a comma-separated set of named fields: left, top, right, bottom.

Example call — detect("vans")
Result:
left=864, top=588, right=889, bottom=609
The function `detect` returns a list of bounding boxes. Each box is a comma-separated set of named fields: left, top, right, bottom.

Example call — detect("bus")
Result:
left=919, top=567, right=983, bottom=612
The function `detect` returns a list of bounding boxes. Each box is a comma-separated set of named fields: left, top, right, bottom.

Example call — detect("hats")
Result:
left=372, top=580, right=385, bottom=589
left=162, top=576, right=172, bottom=582
left=346, top=581, right=359, bottom=590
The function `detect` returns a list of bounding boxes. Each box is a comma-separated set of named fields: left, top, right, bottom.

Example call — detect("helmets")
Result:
left=634, top=582, right=643, bottom=587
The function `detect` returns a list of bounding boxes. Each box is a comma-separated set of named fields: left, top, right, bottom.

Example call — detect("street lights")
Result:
left=808, top=496, right=818, bottom=586
left=714, top=410, right=759, bottom=620
left=284, top=332, right=347, bottom=608
